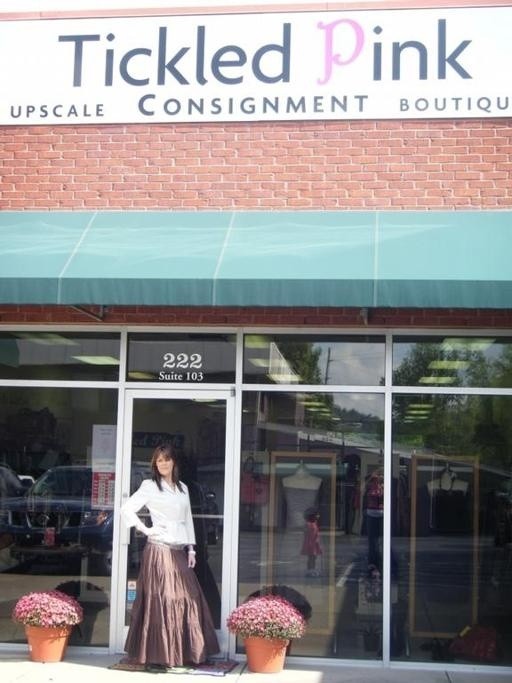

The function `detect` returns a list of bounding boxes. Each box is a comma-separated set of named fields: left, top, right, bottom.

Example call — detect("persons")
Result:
left=281, top=466, right=323, bottom=604
left=427, top=461, right=469, bottom=540
left=240, top=456, right=256, bottom=529
left=120, top=444, right=219, bottom=668
left=363, top=468, right=383, bottom=566
left=341, top=453, right=361, bottom=535
left=301, top=507, right=323, bottom=576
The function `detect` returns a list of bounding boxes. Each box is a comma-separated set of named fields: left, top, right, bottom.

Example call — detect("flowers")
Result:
left=12, top=589, right=86, bottom=626
left=225, top=592, right=309, bottom=640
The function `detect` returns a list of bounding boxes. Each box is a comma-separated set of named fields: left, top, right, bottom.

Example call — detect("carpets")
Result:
left=106, top=654, right=240, bottom=676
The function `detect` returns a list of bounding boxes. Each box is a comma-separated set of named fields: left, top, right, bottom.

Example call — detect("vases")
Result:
left=241, top=636, right=291, bottom=674
left=25, top=623, right=75, bottom=661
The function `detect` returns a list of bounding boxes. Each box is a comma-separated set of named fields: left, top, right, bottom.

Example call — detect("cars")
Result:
left=0, top=450, right=220, bottom=569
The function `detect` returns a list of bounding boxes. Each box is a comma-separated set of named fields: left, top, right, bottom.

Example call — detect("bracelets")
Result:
left=187, top=550, right=196, bottom=556
